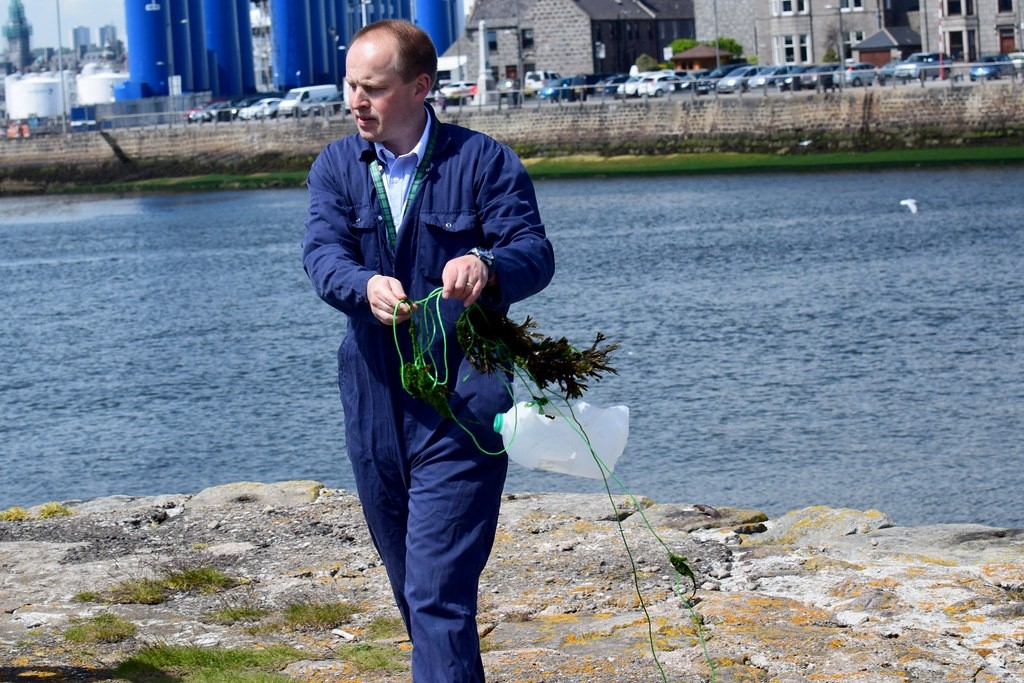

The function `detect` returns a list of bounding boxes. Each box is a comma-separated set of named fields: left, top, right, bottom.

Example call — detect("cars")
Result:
left=1007, top=51, right=1024, bottom=70
left=694, top=64, right=745, bottom=93
left=525, top=71, right=563, bottom=92
left=775, top=65, right=815, bottom=91
left=798, top=63, right=839, bottom=89
left=617, top=70, right=696, bottom=97
left=746, top=65, right=799, bottom=89
left=969, top=55, right=1018, bottom=81
left=716, top=64, right=767, bottom=94
left=187, top=101, right=237, bottom=122
left=434, top=80, right=477, bottom=99
left=593, top=73, right=630, bottom=93
left=238, top=98, right=283, bottom=120
left=211, top=96, right=266, bottom=121
left=877, top=60, right=903, bottom=86
left=536, top=77, right=571, bottom=98
left=572, top=72, right=617, bottom=98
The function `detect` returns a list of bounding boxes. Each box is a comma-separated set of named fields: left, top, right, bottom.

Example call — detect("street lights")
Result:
left=361, top=0, right=373, bottom=28
left=825, top=1, right=846, bottom=87
left=327, top=25, right=346, bottom=81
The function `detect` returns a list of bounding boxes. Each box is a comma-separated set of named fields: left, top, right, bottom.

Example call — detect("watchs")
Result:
left=466, top=247, right=496, bottom=280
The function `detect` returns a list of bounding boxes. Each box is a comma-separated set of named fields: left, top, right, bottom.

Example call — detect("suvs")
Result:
left=894, top=52, right=955, bottom=85
left=831, top=62, right=878, bottom=87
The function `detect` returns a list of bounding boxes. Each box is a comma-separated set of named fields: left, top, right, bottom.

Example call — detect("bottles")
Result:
left=494, top=401, right=629, bottom=480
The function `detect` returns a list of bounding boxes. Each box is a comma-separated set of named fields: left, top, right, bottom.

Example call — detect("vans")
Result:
left=277, top=82, right=339, bottom=118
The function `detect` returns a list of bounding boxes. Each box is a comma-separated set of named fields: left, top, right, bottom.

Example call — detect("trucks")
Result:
left=5, top=114, right=70, bottom=140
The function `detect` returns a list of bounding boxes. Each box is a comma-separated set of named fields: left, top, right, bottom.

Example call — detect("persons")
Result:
left=302, top=22, right=556, bottom=683
left=509, top=72, right=522, bottom=105
